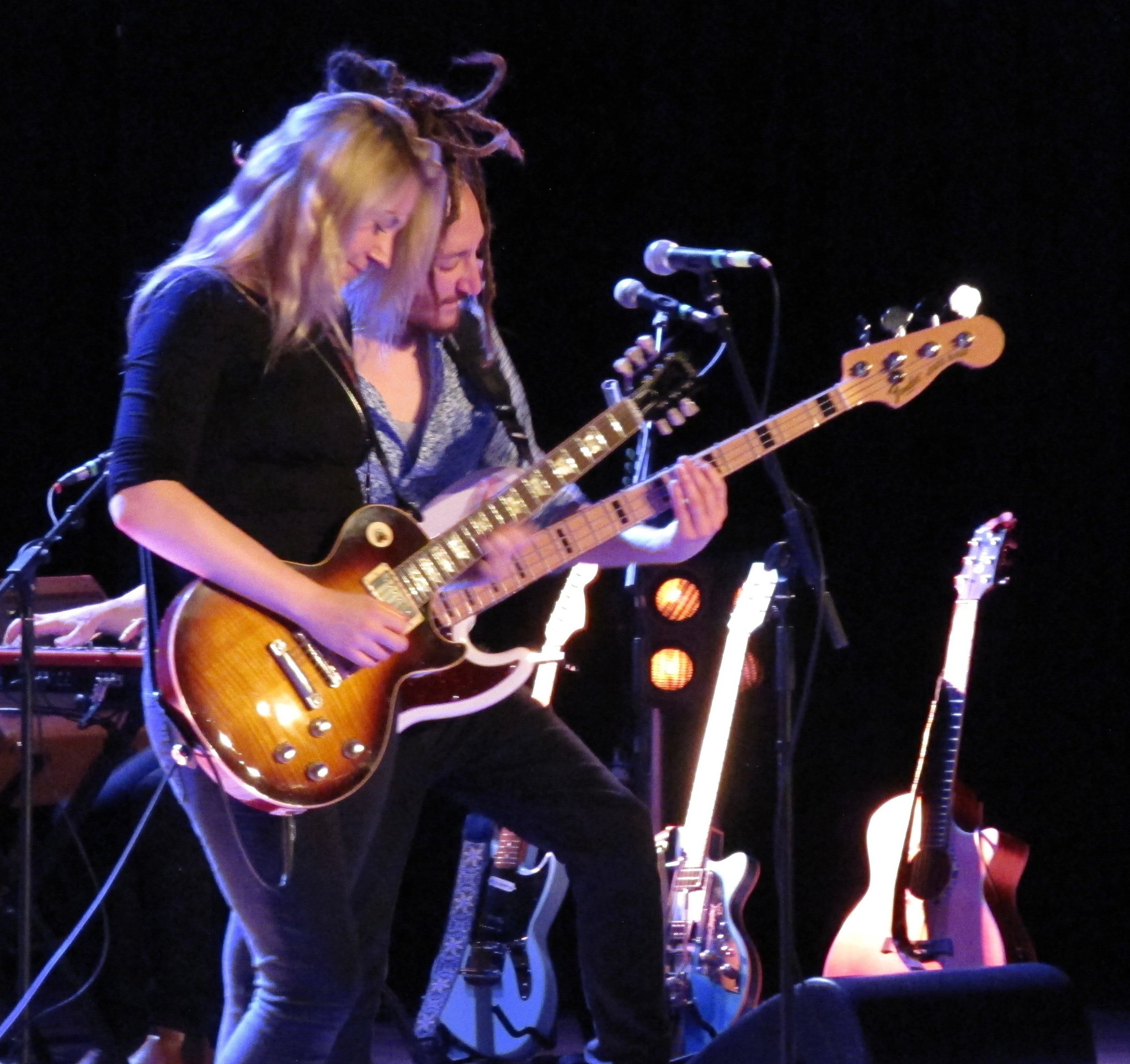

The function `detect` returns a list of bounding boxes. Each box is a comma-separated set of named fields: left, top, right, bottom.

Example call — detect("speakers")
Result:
left=690, top=965, right=1094, bottom=1064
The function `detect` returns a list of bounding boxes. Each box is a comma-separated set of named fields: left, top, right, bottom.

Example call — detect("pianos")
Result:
left=0, top=638, right=151, bottom=1050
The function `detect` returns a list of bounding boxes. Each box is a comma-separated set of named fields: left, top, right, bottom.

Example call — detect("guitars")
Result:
left=395, top=283, right=1007, bottom=742
left=651, top=556, right=798, bottom=1064
left=431, top=559, right=601, bottom=1059
left=149, top=332, right=709, bottom=818
left=819, top=510, right=1035, bottom=980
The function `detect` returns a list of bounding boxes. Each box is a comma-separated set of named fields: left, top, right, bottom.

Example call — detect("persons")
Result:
left=3, top=49, right=726, bottom=1064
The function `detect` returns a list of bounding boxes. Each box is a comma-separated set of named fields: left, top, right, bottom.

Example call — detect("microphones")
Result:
left=613, top=279, right=717, bottom=333
left=55, top=449, right=112, bottom=488
left=643, top=239, right=772, bottom=276
